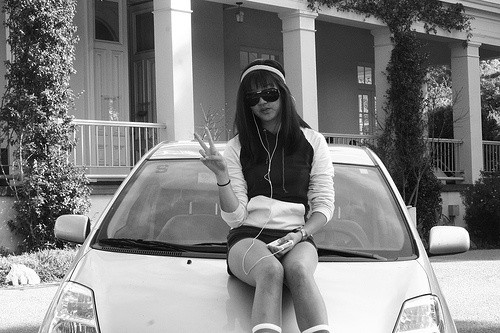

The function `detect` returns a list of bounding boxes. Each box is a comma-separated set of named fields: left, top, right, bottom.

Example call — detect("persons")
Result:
left=194, top=58, right=335, bottom=333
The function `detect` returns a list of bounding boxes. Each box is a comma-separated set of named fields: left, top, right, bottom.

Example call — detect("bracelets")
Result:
left=296, top=226, right=308, bottom=241
left=217, top=179, right=230, bottom=187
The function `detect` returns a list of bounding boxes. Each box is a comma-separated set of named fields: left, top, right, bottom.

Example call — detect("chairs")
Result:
left=312, top=183, right=372, bottom=250
left=155, top=183, right=232, bottom=243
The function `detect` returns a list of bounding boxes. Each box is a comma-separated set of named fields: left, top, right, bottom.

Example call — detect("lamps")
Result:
left=236, top=1, right=245, bottom=23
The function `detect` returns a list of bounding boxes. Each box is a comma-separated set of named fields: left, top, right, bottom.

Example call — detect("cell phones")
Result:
left=267, top=237, right=292, bottom=251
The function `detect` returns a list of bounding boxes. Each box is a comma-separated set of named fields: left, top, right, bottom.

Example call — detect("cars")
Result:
left=38, top=139, right=471, bottom=333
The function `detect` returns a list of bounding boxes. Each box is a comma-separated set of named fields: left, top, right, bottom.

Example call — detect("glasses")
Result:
left=245, top=87, right=282, bottom=105
